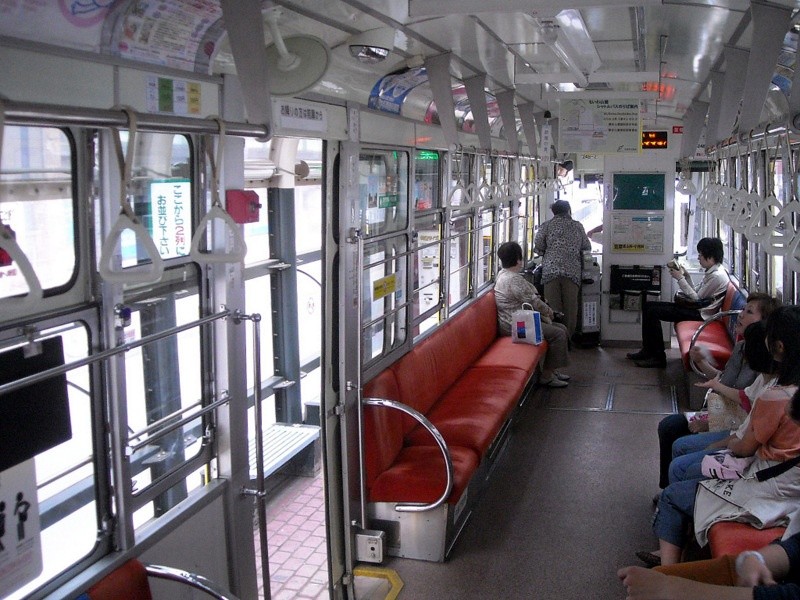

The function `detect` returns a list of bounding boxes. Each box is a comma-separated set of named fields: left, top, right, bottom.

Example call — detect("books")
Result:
left=667, top=259, right=684, bottom=275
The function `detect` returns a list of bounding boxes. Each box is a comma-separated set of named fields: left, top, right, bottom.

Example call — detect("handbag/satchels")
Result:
left=702, top=450, right=755, bottom=480
left=707, top=392, right=748, bottom=431
left=512, top=303, right=544, bottom=345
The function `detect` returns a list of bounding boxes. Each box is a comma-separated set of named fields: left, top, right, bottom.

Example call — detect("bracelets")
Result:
left=735, top=550, right=766, bottom=577
left=678, top=276, right=685, bottom=284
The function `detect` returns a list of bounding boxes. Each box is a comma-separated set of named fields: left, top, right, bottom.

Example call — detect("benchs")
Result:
left=673, top=274, right=750, bottom=411
left=249, top=421, right=321, bottom=481
left=707, top=522, right=786, bottom=558
left=363, top=291, right=548, bottom=563
left=71, top=559, right=242, bottom=600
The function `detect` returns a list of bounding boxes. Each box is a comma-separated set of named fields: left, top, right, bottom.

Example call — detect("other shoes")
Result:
left=555, top=371, right=570, bottom=379
left=637, top=355, right=667, bottom=369
left=628, top=348, right=646, bottom=359
left=637, top=551, right=662, bottom=566
left=541, top=375, right=568, bottom=387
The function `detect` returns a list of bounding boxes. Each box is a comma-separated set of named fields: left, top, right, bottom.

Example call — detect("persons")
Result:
left=494, top=241, right=573, bottom=387
left=558, top=166, right=567, bottom=176
left=535, top=200, right=591, bottom=351
left=617, top=291, right=800, bottom=600
left=627, top=237, right=730, bottom=368
